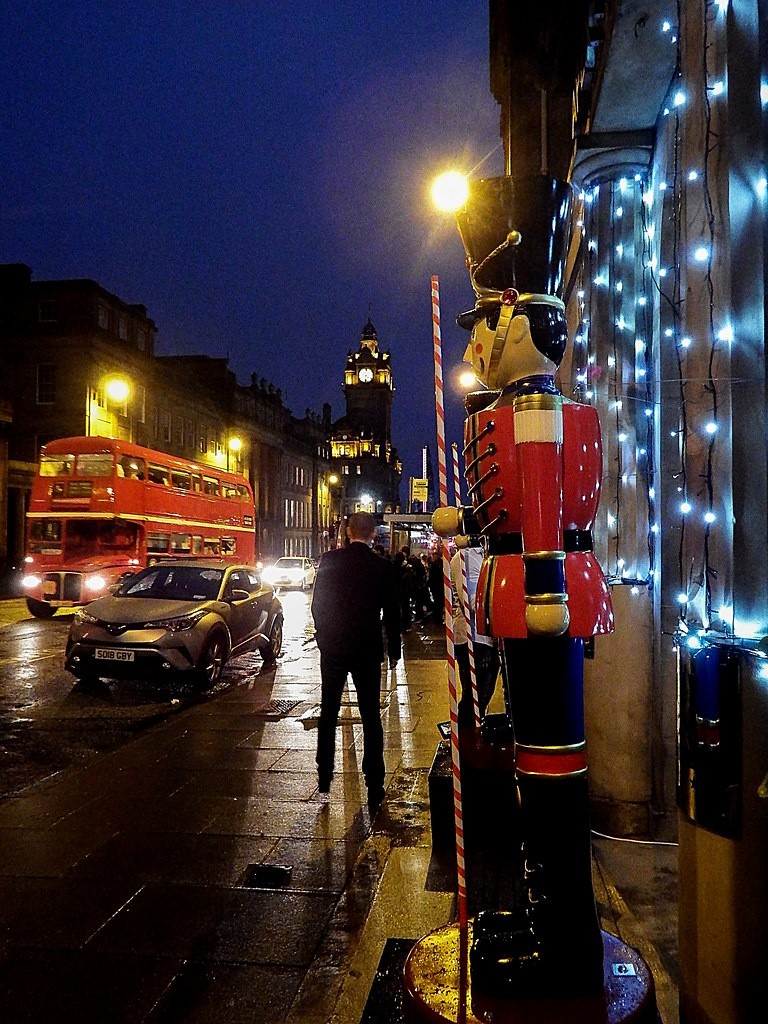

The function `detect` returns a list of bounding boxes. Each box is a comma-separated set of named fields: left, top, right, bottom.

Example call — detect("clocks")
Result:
left=359, top=368, right=374, bottom=383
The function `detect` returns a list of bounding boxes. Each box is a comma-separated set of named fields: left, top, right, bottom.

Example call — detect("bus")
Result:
left=22, top=437, right=256, bottom=618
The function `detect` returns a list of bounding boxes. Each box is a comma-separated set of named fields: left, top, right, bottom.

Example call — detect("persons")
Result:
left=450, top=515, right=500, bottom=718
left=311, top=510, right=401, bottom=806
left=376, top=545, right=445, bottom=669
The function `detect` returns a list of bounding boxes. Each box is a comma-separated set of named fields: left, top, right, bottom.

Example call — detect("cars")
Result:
left=269, top=557, right=316, bottom=592
left=64, top=561, right=284, bottom=688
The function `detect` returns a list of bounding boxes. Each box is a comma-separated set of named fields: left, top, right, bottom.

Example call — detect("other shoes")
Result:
left=316, top=765, right=335, bottom=772
left=367, top=787, right=386, bottom=807
